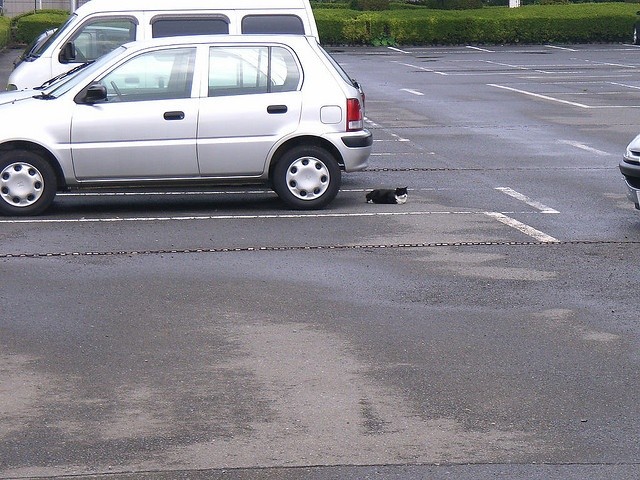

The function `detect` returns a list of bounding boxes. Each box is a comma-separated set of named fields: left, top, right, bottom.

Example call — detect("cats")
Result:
left=366, top=186, right=408, bottom=204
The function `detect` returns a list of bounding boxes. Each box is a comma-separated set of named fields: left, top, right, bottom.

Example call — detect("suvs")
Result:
left=0, top=34, right=372, bottom=217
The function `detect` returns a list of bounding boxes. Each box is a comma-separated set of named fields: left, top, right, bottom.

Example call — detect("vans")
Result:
left=22, top=26, right=132, bottom=62
left=7, top=0, right=321, bottom=91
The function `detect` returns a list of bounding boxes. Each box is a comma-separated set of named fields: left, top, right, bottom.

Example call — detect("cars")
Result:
left=620, top=133, right=640, bottom=210
left=633, top=11, right=640, bottom=45
